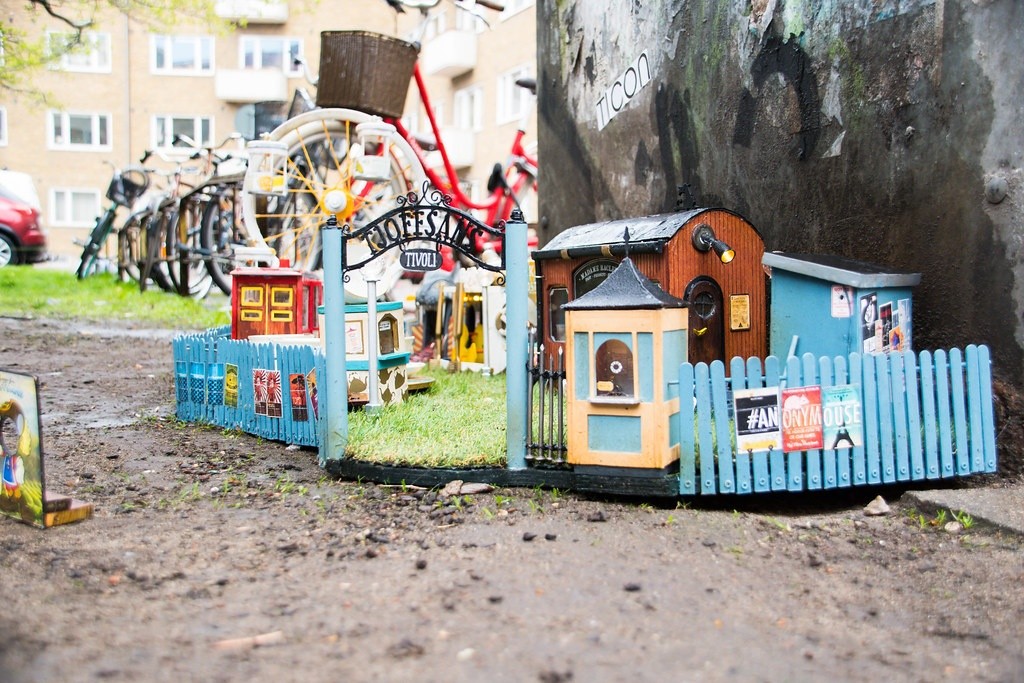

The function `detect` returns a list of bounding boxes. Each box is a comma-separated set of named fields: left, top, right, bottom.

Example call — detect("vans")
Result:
left=0, top=171, right=42, bottom=220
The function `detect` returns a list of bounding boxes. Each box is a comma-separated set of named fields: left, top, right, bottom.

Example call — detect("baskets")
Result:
left=106, top=177, right=141, bottom=209
left=315, top=30, right=417, bottom=119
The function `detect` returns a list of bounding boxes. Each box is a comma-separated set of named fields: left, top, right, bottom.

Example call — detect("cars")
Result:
left=1, top=193, right=46, bottom=269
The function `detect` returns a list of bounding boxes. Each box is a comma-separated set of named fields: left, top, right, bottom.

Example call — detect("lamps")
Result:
left=700, top=234, right=735, bottom=263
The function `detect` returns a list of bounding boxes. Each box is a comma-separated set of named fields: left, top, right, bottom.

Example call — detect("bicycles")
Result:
left=242, top=0, right=541, bottom=280
left=73, top=133, right=321, bottom=303
